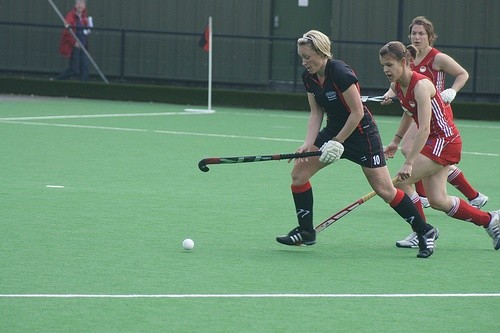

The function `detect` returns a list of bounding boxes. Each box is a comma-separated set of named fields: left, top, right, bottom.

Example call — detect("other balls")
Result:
left=183, top=239, right=193, bottom=250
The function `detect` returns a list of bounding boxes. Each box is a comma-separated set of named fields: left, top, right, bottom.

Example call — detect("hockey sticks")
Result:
left=361, top=94, right=400, bottom=103
left=316, top=176, right=400, bottom=234
left=198, top=149, right=322, bottom=172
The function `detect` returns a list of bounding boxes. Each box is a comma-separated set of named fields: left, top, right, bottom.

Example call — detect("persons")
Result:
left=378, top=41, right=500, bottom=250
left=58, top=0, right=94, bottom=81
left=275, top=29, right=440, bottom=258
left=380, top=16, right=489, bottom=211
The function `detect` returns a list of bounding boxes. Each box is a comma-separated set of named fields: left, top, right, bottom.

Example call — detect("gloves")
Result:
left=319, top=139, right=344, bottom=163
left=441, top=89, right=457, bottom=103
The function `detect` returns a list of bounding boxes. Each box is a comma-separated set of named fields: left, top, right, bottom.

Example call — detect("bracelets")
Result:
left=332, top=136, right=345, bottom=144
left=395, top=134, right=402, bottom=139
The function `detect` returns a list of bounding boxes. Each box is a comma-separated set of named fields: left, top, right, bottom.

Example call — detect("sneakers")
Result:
left=276, top=226, right=317, bottom=245
left=396, top=232, right=420, bottom=248
left=419, top=195, right=430, bottom=208
left=468, top=192, right=489, bottom=209
left=483, top=208, right=500, bottom=250
left=416, top=223, right=439, bottom=257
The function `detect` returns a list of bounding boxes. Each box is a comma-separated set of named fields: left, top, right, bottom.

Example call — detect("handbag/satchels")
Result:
left=59, top=29, right=73, bottom=56
